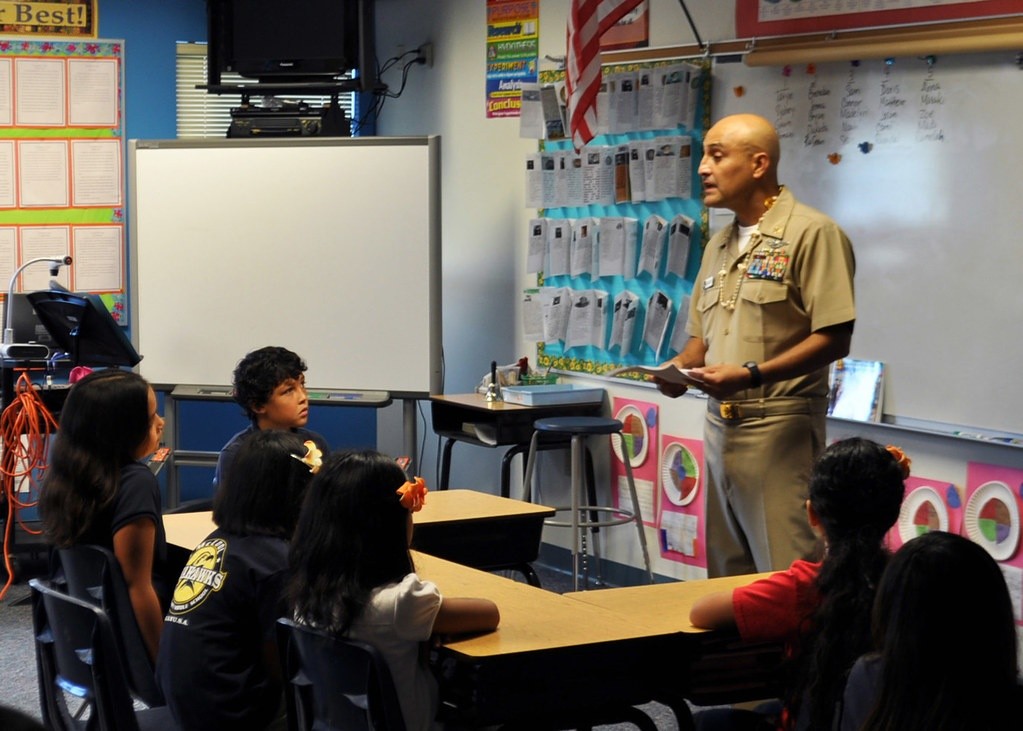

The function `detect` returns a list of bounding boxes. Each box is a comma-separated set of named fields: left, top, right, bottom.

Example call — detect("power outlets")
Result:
left=417, top=43, right=433, bottom=70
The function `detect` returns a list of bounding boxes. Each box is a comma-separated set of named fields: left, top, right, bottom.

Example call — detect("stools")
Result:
left=519, top=416, right=655, bottom=593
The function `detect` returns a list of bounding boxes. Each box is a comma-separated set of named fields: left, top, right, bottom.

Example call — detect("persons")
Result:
left=155, top=427, right=500, bottom=731
left=688, top=437, right=1023, bottom=731
left=36, top=370, right=173, bottom=708
left=652, top=114, right=857, bottom=579
left=213, top=346, right=333, bottom=489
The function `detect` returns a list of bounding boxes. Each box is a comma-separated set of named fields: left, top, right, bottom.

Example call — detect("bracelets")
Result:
left=743, top=360, right=762, bottom=390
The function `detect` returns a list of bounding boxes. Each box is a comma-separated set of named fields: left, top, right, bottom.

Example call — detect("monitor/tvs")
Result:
left=226, top=0, right=359, bottom=84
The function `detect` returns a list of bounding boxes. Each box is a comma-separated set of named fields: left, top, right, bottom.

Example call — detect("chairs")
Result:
left=70, top=545, right=166, bottom=723
left=276, top=617, right=407, bottom=731
left=27, top=579, right=179, bottom=731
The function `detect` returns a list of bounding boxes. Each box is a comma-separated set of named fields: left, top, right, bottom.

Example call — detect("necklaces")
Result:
left=717, top=184, right=782, bottom=311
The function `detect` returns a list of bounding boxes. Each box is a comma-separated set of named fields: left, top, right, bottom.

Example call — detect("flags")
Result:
left=564, top=0, right=645, bottom=156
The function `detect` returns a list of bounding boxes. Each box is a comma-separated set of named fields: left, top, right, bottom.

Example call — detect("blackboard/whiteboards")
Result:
left=129, top=134, right=444, bottom=401
left=708, top=50, right=1023, bottom=434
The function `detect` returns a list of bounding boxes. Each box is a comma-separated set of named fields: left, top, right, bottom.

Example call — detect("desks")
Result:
left=0, top=356, right=72, bottom=588
left=428, top=394, right=604, bottom=587
left=162, top=489, right=787, bottom=731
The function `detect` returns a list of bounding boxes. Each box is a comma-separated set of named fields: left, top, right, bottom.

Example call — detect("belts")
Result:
left=707, top=395, right=830, bottom=421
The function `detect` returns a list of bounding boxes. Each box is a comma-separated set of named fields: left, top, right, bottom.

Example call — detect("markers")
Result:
left=953, top=431, right=992, bottom=442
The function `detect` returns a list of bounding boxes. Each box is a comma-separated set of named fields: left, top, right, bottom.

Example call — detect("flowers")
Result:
left=397, top=476, right=428, bottom=513
left=885, top=444, right=911, bottom=480
left=291, top=440, right=322, bottom=474
